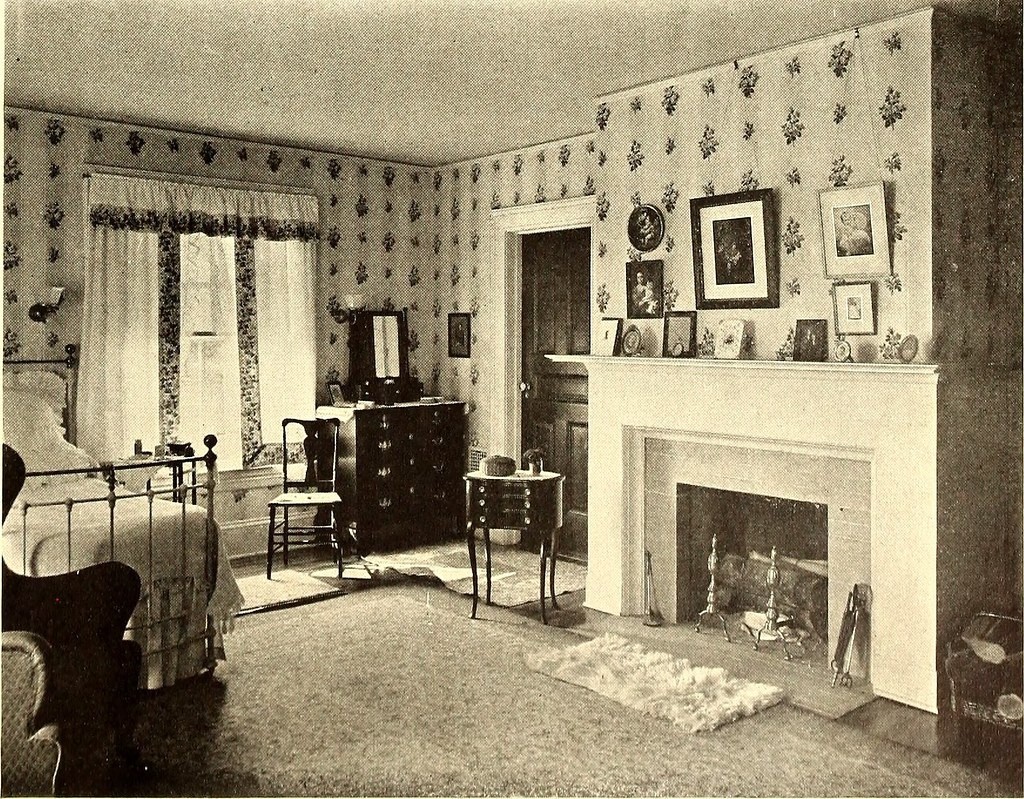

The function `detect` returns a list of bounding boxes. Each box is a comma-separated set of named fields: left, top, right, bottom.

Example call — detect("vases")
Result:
left=529, top=460, right=543, bottom=475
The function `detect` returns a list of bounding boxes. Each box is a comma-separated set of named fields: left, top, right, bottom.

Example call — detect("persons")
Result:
left=840, top=212, right=871, bottom=244
left=631, top=270, right=656, bottom=315
left=455, top=324, right=464, bottom=346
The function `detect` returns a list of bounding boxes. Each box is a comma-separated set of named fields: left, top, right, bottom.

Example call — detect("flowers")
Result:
left=523, top=448, right=547, bottom=464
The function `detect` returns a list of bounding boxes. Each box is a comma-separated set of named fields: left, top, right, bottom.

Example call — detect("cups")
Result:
left=155, top=446, right=166, bottom=461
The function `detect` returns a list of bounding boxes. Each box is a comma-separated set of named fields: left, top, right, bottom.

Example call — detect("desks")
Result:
left=116, top=456, right=203, bottom=504
left=463, top=467, right=566, bottom=626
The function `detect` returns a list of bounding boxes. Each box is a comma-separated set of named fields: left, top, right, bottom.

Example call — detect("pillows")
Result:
left=2, top=371, right=102, bottom=489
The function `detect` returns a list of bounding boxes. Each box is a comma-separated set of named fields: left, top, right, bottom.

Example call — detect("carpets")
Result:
left=227, top=568, right=340, bottom=616
left=360, top=536, right=586, bottom=607
left=126, top=591, right=1022, bottom=797
left=525, top=632, right=784, bottom=733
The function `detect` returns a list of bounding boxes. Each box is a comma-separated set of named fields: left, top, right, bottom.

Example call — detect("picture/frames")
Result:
left=663, top=311, right=697, bottom=359
left=627, top=203, right=665, bottom=252
left=715, top=319, right=744, bottom=359
left=690, top=188, right=780, bottom=311
left=625, top=260, right=664, bottom=319
left=448, top=312, right=471, bottom=358
left=326, top=381, right=346, bottom=407
left=817, top=181, right=892, bottom=280
left=792, top=319, right=829, bottom=363
left=832, top=280, right=878, bottom=336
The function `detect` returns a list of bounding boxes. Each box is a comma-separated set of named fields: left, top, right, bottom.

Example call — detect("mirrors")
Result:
left=350, top=309, right=409, bottom=379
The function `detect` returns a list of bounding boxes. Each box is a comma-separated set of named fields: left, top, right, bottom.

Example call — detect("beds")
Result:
left=2, top=344, right=245, bottom=696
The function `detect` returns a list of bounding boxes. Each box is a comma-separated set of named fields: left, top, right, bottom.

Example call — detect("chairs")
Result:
left=267, top=418, right=344, bottom=580
left=2, top=443, right=157, bottom=797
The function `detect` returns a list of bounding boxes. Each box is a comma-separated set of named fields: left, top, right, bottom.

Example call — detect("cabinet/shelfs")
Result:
left=316, top=400, right=466, bottom=559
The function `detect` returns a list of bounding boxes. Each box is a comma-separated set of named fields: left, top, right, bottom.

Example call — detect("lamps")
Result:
left=28, top=287, right=67, bottom=324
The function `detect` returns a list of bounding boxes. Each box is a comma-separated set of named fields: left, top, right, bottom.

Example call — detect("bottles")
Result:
left=384, top=376, right=396, bottom=406
left=135, top=439, right=142, bottom=456
